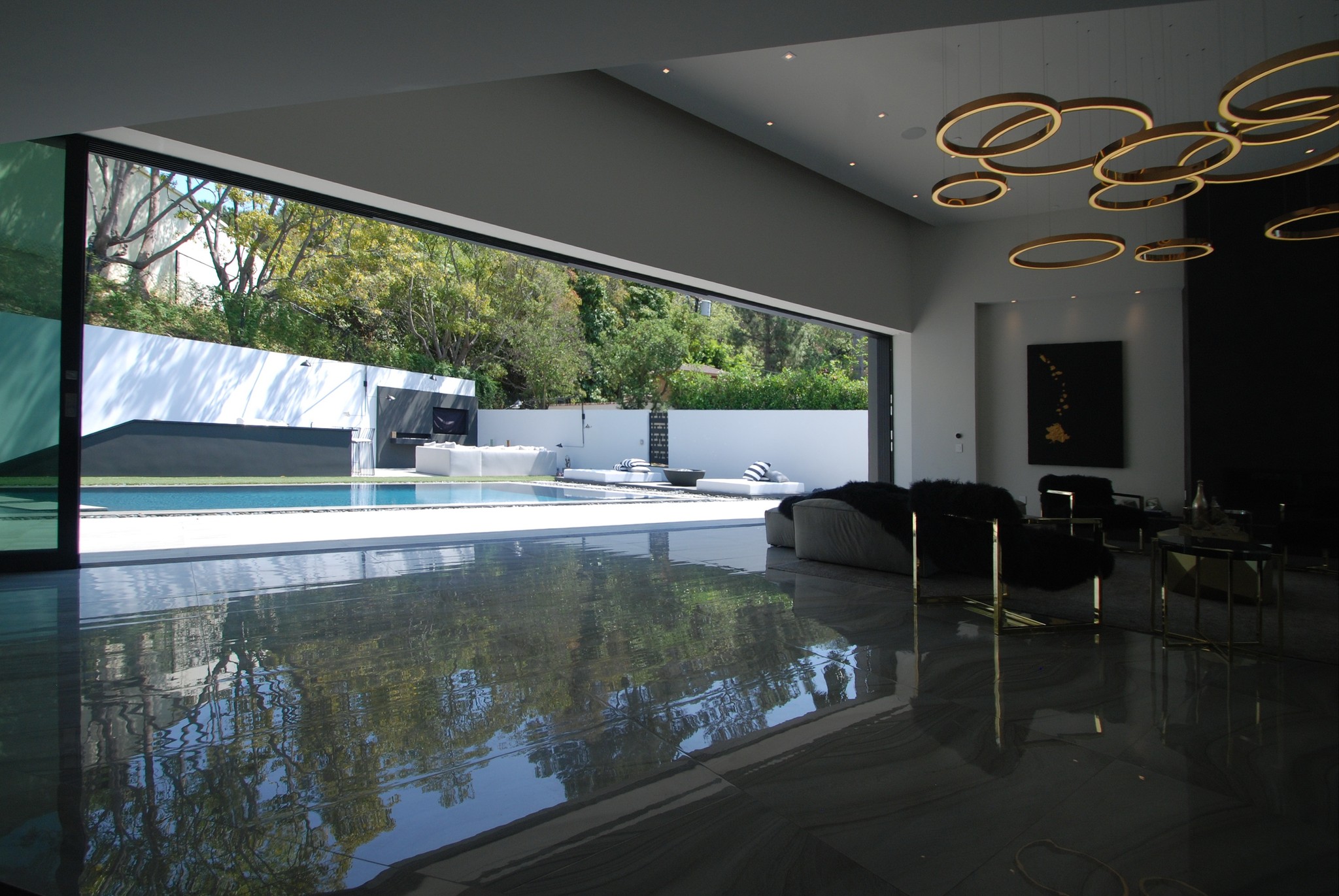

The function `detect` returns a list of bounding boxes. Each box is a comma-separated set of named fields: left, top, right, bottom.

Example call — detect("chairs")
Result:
left=1038, top=474, right=1144, bottom=554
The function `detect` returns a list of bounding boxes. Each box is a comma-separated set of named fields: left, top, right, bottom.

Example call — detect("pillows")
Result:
left=620, top=458, right=650, bottom=468
left=743, top=461, right=790, bottom=483
left=613, top=463, right=651, bottom=473
left=424, top=441, right=546, bottom=450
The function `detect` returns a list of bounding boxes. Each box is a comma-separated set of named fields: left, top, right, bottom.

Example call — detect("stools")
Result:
left=331, top=426, right=375, bottom=475
left=765, top=508, right=795, bottom=547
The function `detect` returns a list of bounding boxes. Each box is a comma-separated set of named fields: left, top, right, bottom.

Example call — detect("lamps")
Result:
left=300, top=360, right=437, bottom=381
left=556, top=443, right=583, bottom=448
left=367, top=394, right=396, bottom=401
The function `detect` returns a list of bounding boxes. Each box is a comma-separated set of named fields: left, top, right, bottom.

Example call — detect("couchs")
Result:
left=415, top=445, right=557, bottom=477
left=792, top=499, right=921, bottom=576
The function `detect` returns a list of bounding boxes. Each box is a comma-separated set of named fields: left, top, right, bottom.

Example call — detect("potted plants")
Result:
left=562, top=455, right=573, bottom=473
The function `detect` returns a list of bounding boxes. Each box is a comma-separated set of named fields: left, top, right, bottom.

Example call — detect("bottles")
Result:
left=1192, top=479, right=1208, bottom=530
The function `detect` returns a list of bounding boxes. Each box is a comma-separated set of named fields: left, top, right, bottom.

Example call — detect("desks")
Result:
left=1149, top=528, right=1283, bottom=663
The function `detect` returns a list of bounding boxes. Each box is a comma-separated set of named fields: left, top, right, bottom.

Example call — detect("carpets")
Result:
left=767, top=551, right=1339, bottom=666
